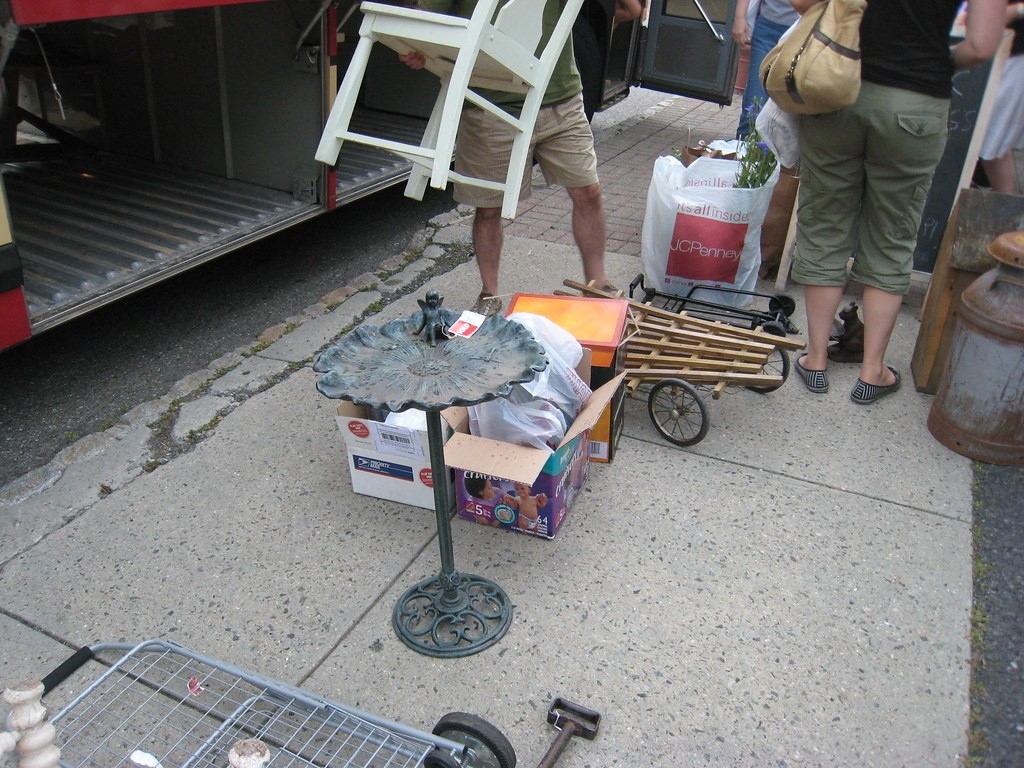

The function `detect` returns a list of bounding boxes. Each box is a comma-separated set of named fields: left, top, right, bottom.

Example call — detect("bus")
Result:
left=1, top=2, right=748, bottom=351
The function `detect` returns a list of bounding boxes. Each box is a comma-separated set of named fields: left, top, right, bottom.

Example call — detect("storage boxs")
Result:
left=439, top=346, right=629, bottom=541
left=504, top=293, right=629, bottom=464
left=335, top=400, right=456, bottom=512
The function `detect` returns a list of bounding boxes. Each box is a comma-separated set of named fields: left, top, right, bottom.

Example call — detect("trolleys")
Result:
left=8, top=638, right=517, bottom=768
left=629, top=273, right=800, bottom=448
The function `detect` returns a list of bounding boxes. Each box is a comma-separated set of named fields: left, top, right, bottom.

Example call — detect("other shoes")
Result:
left=469, top=291, right=502, bottom=318
left=583, top=283, right=645, bottom=318
left=850, top=365, right=901, bottom=404
left=794, top=352, right=829, bottom=392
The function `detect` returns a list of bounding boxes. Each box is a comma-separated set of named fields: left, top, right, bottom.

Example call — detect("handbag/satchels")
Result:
left=759, top=0, right=869, bottom=115
left=754, top=97, right=802, bottom=169
left=744, top=0, right=760, bottom=44
left=467, top=312, right=591, bottom=452
left=640, top=138, right=799, bottom=309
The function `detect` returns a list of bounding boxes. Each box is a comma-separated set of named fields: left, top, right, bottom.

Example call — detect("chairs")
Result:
left=314, top=0, right=583, bottom=221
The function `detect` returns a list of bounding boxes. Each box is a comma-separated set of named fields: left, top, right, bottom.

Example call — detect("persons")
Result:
left=400, top=0, right=641, bottom=318
left=732, top=0, right=799, bottom=143
left=787, top=0, right=1007, bottom=405
left=957, top=0, right=1024, bottom=195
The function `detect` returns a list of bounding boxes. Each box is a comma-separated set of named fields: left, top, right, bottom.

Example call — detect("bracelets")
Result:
left=1017, top=4, right=1024, bottom=16
